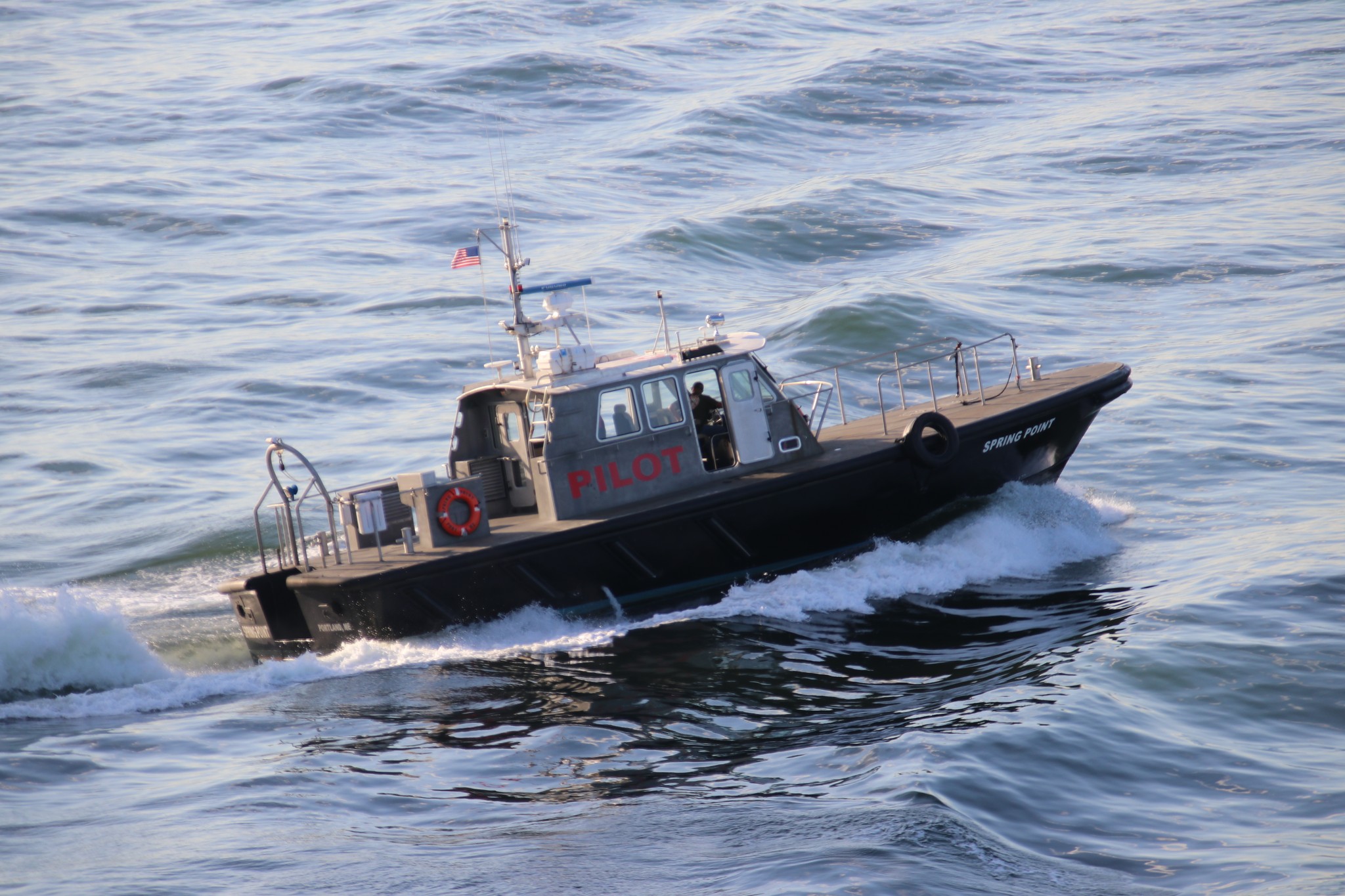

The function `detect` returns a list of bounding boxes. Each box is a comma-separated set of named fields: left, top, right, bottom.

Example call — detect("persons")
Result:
left=669, top=381, right=724, bottom=454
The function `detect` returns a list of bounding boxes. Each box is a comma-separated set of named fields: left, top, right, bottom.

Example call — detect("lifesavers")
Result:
left=438, top=488, right=480, bottom=537
left=904, top=411, right=958, bottom=469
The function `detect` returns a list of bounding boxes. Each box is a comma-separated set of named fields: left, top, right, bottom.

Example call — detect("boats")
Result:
left=216, top=141, right=1130, bottom=666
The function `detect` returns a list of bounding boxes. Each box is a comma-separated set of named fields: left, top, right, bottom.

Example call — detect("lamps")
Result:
left=656, top=290, right=662, bottom=299
left=705, top=314, right=725, bottom=337
left=503, top=217, right=508, bottom=225
left=524, top=257, right=530, bottom=265
left=498, top=320, right=508, bottom=329
left=513, top=361, right=522, bottom=376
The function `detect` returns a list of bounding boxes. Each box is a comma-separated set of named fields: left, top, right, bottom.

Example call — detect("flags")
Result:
left=451, top=245, right=481, bottom=268
